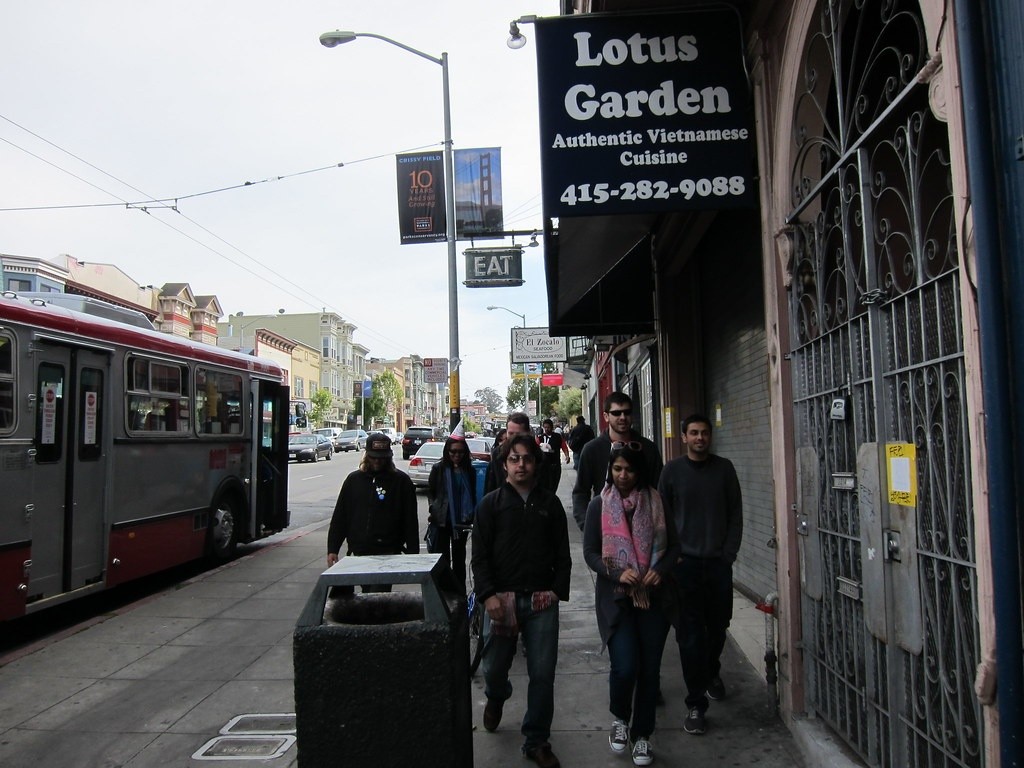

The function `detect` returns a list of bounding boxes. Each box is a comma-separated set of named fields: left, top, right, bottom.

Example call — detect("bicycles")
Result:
left=451, top=523, right=485, bottom=679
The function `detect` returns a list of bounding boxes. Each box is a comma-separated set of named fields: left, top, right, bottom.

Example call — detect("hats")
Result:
left=366, top=432, right=392, bottom=457
left=450, top=417, right=466, bottom=441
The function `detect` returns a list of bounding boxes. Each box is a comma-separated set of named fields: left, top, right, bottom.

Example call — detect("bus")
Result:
left=0, top=291, right=307, bottom=624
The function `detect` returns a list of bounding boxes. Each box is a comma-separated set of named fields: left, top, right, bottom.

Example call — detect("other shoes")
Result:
left=521, top=737, right=562, bottom=768
left=483, top=699, right=505, bottom=732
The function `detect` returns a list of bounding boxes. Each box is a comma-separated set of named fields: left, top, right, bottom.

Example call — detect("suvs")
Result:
left=311, top=427, right=343, bottom=447
left=401, top=426, right=451, bottom=460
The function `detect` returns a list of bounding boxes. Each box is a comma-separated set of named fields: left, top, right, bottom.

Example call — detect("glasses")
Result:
left=507, top=454, right=537, bottom=465
left=608, top=409, right=633, bottom=416
left=610, top=440, right=642, bottom=453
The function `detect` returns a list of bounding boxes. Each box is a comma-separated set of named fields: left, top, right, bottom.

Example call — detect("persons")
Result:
left=327, top=433, right=421, bottom=593
left=483, top=412, right=530, bottom=495
left=555, top=426, right=563, bottom=434
left=426, top=418, right=477, bottom=578
left=569, top=416, right=595, bottom=471
left=535, top=418, right=570, bottom=495
left=571, top=392, right=664, bottom=533
left=471, top=434, right=572, bottom=768
left=563, top=424, right=569, bottom=443
left=657, top=414, right=743, bottom=734
left=583, top=442, right=682, bottom=765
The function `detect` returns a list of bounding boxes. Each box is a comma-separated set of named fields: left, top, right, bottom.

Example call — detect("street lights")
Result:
left=319, top=28, right=461, bottom=435
left=240, top=313, right=279, bottom=353
left=487, top=305, right=529, bottom=412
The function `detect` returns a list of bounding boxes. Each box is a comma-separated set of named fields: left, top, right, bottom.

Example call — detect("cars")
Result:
left=395, top=432, right=404, bottom=444
left=334, top=429, right=369, bottom=453
left=465, top=416, right=508, bottom=462
left=406, top=441, right=446, bottom=488
left=263, top=431, right=333, bottom=463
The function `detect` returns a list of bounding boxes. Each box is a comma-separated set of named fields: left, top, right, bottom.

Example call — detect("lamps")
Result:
left=529, top=232, right=543, bottom=246
left=506, top=14, right=537, bottom=49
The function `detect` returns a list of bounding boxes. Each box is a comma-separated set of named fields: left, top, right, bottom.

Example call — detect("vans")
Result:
left=366, top=427, right=397, bottom=446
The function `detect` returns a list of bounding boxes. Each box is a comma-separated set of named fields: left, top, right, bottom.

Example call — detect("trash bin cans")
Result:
left=292, top=552, right=474, bottom=768
left=471, top=461, right=489, bottom=504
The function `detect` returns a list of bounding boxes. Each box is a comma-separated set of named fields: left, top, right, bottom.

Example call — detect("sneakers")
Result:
left=685, top=706, right=706, bottom=733
left=608, top=720, right=632, bottom=753
left=629, top=736, right=654, bottom=765
left=706, top=677, right=726, bottom=700
